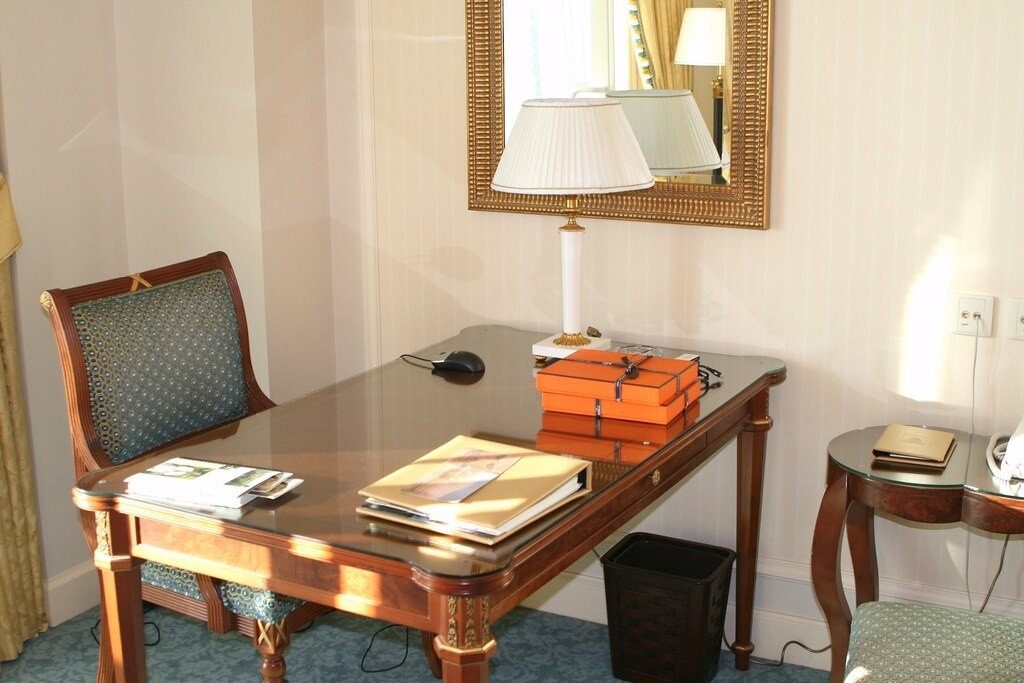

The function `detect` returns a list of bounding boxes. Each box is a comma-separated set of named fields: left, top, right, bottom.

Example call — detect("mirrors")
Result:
left=465, top=0, right=774, bottom=232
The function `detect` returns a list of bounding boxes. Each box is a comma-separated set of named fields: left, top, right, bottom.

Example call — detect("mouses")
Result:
left=430, top=351, right=484, bottom=374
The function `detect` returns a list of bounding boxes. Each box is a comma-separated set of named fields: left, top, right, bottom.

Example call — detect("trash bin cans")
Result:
left=600, top=531, right=737, bottom=683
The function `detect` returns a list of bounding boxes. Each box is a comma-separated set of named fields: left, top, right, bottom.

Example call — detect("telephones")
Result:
left=998, top=411, right=1024, bottom=479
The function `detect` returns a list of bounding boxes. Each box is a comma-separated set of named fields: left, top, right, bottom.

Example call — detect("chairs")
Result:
left=39, top=249, right=442, bottom=683
left=844, top=602, right=1024, bottom=683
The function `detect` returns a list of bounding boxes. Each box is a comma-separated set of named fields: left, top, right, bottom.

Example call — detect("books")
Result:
left=873, top=420, right=957, bottom=469
left=356, top=434, right=591, bottom=546
left=123, top=456, right=304, bottom=521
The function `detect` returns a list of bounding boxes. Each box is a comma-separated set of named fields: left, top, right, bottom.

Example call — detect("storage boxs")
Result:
left=542, top=379, right=702, bottom=425
left=536, top=349, right=699, bottom=407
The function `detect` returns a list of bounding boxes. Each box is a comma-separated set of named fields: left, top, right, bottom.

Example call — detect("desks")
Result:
left=71, top=322, right=787, bottom=683
left=809, top=425, right=1024, bottom=683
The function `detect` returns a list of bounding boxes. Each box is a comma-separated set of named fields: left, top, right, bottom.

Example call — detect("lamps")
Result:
left=605, top=88, right=722, bottom=183
left=673, top=1, right=730, bottom=185
left=488, top=96, right=657, bottom=370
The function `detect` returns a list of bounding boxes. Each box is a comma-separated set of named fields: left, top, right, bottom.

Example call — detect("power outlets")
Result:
left=951, top=292, right=995, bottom=337
